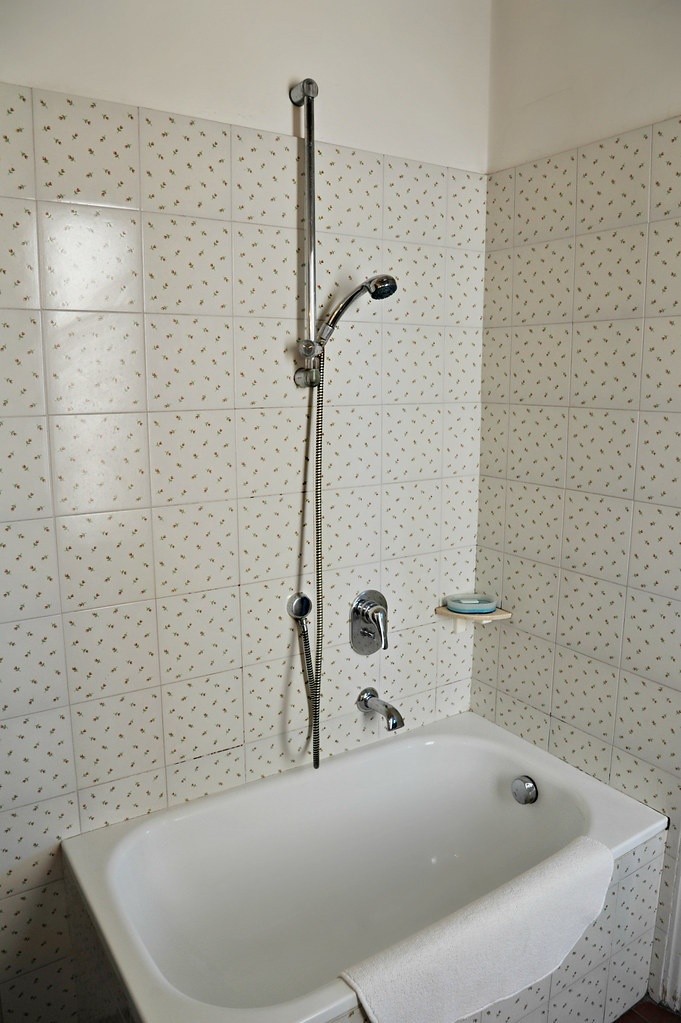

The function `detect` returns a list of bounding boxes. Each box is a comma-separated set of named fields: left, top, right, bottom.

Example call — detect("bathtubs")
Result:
left=59, top=703, right=671, bottom=1023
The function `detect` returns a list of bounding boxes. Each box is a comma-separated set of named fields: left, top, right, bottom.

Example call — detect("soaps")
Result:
left=460, top=597, right=480, bottom=605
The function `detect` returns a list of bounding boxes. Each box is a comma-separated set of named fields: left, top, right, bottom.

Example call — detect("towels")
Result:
left=338, top=834, right=615, bottom=1023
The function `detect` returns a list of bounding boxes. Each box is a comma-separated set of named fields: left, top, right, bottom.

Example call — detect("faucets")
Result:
left=358, top=687, right=407, bottom=733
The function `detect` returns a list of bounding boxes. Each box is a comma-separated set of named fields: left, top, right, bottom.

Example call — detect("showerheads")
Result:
left=318, top=275, right=399, bottom=347
